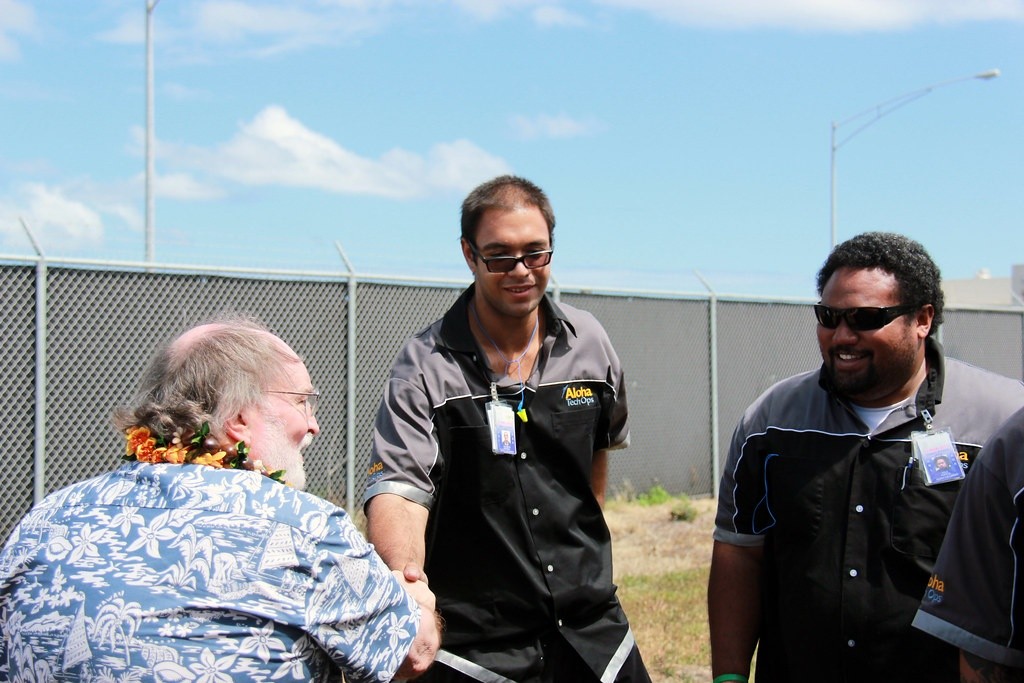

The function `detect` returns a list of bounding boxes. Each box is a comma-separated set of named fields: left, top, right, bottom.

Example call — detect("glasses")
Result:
left=812, top=300, right=918, bottom=332
left=259, top=384, right=319, bottom=416
left=463, top=234, right=553, bottom=273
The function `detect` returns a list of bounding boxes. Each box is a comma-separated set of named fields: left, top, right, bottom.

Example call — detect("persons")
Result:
left=1, top=320, right=439, bottom=683
left=502, top=432, right=511, bottom=446
left=934, top=456, right=955, bottom=478
left=364, top=174, right=651, bottom=683
left=707, top=233, right=1024, bottom=683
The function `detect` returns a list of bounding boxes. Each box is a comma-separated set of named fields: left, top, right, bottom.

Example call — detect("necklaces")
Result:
left=468, top=299, right=539, bottom=421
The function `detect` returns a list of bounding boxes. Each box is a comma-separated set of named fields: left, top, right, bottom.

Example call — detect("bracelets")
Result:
left=712, top=674, right=748, bottom=682
